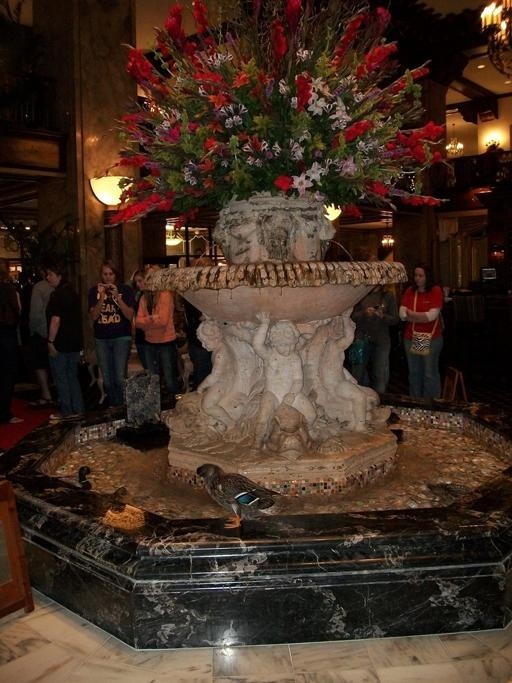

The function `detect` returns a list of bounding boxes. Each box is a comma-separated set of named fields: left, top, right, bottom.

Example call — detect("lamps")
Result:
left=380, top=223, right=394, bottom=248
left=89, top=175, right=138, bottom=208
left=164, top=225, right=184, bottom=247
left=477, top=0, right=511, bottom=86
left=445, top=122, right=464, bottom=157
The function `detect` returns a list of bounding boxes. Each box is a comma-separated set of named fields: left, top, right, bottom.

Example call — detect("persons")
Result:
left=130, top=269, right=156, bottom=370
left=85, top=258, right=137, bottom=411
left=308, top=304, right=393, bottom=435
left=345, top=283, right=401, bottom=394
left=195, top=319, right=240, bottom=432
left=0, top=250, right=86, bottom=426
left=391, top=260, right=446, bottom=402
left=180, top=256, right=218, bottom=390
left=130, top=263, right=182, bottom=409
left=248, top=310, right=317, bottom=455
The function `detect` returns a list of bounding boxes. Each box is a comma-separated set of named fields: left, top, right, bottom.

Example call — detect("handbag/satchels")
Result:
left=410, top=331, right=432, bottom=355
left=347, top=335, right=377, bottom=366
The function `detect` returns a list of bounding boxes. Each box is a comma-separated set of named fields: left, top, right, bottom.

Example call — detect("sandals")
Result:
left=23, top=397, right=56, bottom=409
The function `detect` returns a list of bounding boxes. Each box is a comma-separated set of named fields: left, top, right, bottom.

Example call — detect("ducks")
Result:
left=59, top=466, right=93, bottom=491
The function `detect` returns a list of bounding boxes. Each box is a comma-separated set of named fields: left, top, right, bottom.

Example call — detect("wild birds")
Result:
left=196, top=463, right=283, bottom=530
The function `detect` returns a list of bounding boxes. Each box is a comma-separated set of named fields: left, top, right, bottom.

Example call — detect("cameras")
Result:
left=104, top=284, right=113, bottom=296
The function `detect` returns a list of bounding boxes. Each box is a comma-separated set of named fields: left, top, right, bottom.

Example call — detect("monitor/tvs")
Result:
left=480, top=266, right=497, bottom=282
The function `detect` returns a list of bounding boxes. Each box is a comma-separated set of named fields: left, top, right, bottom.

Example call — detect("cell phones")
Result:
left=374, top=305, right=380, bottom=310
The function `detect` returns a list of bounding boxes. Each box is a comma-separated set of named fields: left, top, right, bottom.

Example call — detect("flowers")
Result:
left=93, top=0, right=460, bottom=238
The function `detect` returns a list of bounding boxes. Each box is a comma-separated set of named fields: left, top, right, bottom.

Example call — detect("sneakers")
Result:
left=49, top=413, right=79, bottom=420
